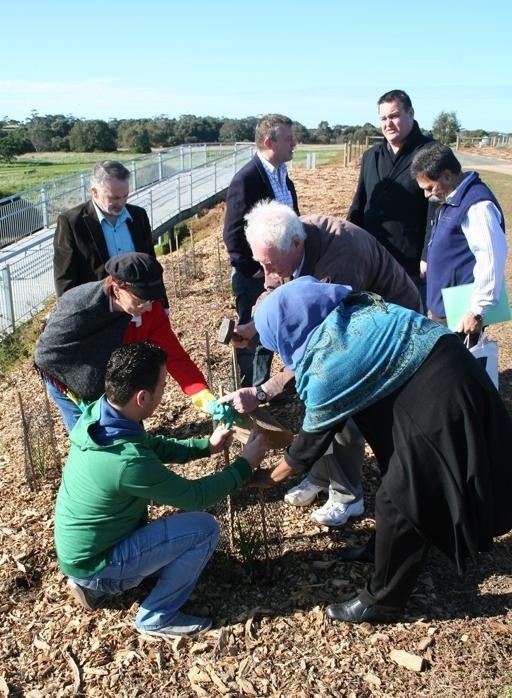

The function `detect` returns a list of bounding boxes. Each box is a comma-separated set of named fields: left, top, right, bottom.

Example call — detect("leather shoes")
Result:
left=326, top=597, right=401, bottom=624
left=337, top=544, right=375, bottom=563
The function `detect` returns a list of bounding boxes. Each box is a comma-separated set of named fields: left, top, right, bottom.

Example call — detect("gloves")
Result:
left=203, top=399, right=243, bottom=429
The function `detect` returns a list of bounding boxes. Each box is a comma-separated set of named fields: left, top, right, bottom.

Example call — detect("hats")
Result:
left=104, top=252, right=163, bottom=300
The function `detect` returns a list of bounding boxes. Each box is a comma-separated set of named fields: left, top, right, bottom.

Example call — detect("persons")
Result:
left=411, top=144, right=509, bottom=348
left=221, top=200, right=423, bottom=526
left=245, top=276, right=512, bottom=623
left=33, top=251, right=219, bottom=431
left=346, top=90, right=440, bottom=317
left=53, top=158, right=169, bottom=316
left=224, top=113, right=300, bottom=405
left=53, top=341, right=266, bottom=640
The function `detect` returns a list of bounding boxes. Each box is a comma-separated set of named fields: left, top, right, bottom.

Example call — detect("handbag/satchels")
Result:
left=464, top=331, right=498, bottom=391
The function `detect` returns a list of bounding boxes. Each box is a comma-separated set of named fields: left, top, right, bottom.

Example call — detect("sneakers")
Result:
left=67, top=579, right=107, bottom=611
left=135, top=612, right=212, bottom=640
left=311, top=496, right=364, bottom=526
left=284, top=478, right=329, bottom=506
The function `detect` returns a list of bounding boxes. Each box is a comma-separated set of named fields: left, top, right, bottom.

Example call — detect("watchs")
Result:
left=253, top=382, right=266, bottom=404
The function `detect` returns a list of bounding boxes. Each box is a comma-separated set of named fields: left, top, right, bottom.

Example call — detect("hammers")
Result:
left=217, top=318, right=256, bottom=350
